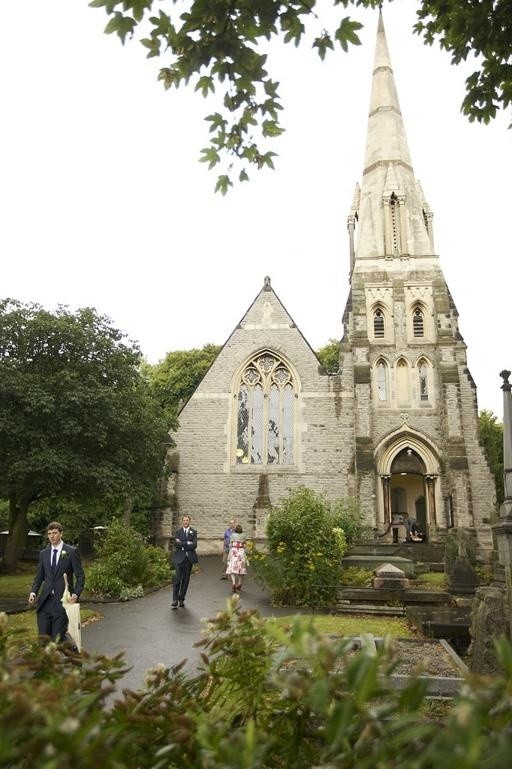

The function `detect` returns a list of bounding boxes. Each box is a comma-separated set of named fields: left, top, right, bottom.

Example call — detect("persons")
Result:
left=227, top=525, right=248, bottom=592
left=28, top=521, right=85, bottom=649
left=170, top=514, right=200, bottom=608
left=219, top=519, right=235, bottom=581
left=412, top=519, right=425, bottom=542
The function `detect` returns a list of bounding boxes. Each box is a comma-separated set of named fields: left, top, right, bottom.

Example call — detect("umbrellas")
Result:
left=1, top=525, right=43, bottom=540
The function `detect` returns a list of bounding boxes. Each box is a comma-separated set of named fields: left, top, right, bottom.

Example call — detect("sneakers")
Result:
left=220, top=576, right=228, bottom=580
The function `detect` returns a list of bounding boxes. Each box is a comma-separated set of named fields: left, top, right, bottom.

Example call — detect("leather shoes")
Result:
left=171, top=601, right=178, bottom=606
left=232, top=583, right=242, bottom=592
left=179, top=599, right=184, bottom=607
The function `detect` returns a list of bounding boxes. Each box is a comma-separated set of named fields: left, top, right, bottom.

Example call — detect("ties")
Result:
left=52, top=549, right=58, bottom=573
left=185, top=530, right=187, bottom=539
